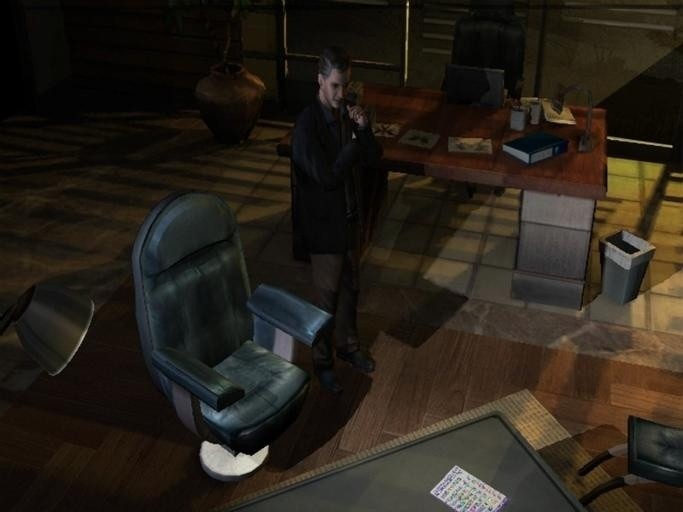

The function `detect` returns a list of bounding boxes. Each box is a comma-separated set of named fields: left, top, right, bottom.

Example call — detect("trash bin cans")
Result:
left=599, top=229, right=657, bottom=305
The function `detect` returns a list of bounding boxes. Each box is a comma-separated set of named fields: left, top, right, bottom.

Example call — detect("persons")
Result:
left=292, top=53, right=376, bottom=384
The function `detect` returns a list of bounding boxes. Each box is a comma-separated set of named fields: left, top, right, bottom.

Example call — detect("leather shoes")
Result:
left=313, top=367, right=343, bottom=396
left=335, top=344, right=375, bottom=372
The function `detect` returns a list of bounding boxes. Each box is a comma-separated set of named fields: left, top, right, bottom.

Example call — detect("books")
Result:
left=502, top=133, right=568, bottom=165
